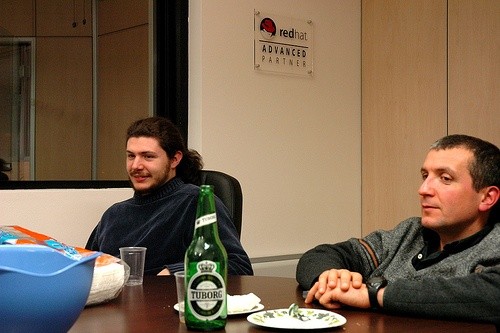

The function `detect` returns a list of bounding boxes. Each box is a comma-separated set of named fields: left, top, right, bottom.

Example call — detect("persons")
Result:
left=84, top=116, right=254, bottom=277
left=295, top=133, right=500, bottom=326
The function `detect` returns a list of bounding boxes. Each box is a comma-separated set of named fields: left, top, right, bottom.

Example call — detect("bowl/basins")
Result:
left=0, top=243, right=103, bottom=333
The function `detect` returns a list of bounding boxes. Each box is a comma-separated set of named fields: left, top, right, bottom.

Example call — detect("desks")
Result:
left=68, top=275, right=500, bottom=333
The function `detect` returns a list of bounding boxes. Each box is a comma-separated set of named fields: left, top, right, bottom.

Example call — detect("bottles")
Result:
left=185, top=185, right=228, bottom=333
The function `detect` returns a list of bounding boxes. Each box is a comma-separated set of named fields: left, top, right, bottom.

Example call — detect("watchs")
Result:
left=366, top=274, right=387, bottom=312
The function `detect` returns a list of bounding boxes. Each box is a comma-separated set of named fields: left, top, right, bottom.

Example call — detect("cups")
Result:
left=119, top=247, right=147, bottom=285
left=174, top=271, right=186, bottom=323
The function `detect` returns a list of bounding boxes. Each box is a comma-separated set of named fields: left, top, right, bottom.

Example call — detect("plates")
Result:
left=246, top=309, right=347, bottom=331
left=173, top=301, right=265, bottom=315
